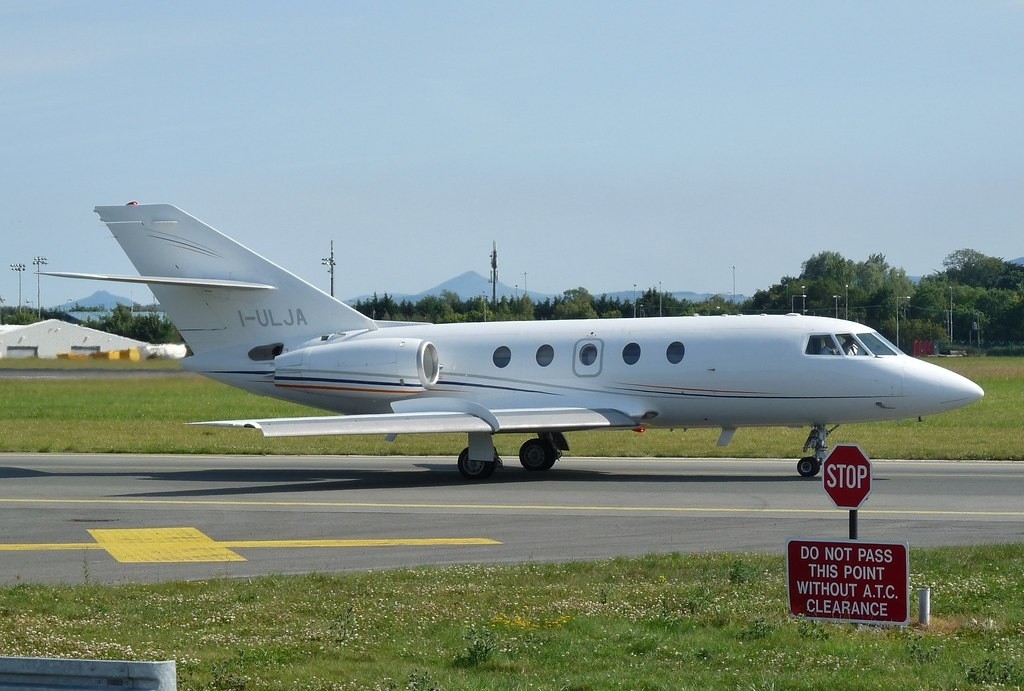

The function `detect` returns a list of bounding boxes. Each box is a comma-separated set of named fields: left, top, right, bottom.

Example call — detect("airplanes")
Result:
left=35, top=197, right=986, bottom=484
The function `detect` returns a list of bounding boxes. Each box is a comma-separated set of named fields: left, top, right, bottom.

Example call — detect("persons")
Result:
left=820, top=338, right=839, bottom=356
left=841, top=338, right=859, bottom=356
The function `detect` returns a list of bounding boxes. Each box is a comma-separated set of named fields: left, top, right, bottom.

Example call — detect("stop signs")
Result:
left=821, top=444, right=872, bottom=511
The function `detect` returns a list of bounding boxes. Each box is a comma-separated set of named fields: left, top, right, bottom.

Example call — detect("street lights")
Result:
left=833, top=295, right=842, bottom=319
left=32, top=256, right=50, bottom=319
left=896, top=296, right=911, bottom=348
left=845, top=285, right=849, bottom=320
left=792, top=295, right=806, bottom=313
left=634, top=284, right=637, bottom=318
left=10, top=264, right=27, bottom=312
left=658, top=282, right=663, bottom=317
left=323, top=240, right=336, bottom=297
left=947, top=285, right=954, bottom=343
left=801, top=285, right=807, bottom=315
